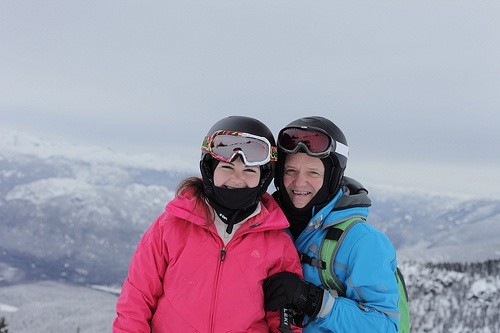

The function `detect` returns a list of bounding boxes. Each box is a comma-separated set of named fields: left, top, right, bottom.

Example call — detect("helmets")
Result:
left=199, top=116, right=277, bottom=199
left=272, top=114, right=350, bottom=197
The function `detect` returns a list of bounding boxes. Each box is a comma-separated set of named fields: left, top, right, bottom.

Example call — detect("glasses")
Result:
left=200, top=130, right=279, bottom=167
left=277, top=124, right=349, bottom=159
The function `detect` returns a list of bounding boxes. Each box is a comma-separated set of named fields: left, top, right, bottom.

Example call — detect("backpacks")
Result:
left=318, top=216, right=412, bottom=332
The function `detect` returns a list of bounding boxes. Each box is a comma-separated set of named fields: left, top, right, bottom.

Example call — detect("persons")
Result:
left=111, top=117, right=304, bottom=333
left=263, top=116, right=399, bottom=333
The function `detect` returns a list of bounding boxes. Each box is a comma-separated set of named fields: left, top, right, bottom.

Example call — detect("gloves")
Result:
left=262, top=271, right=324, bottom=322
